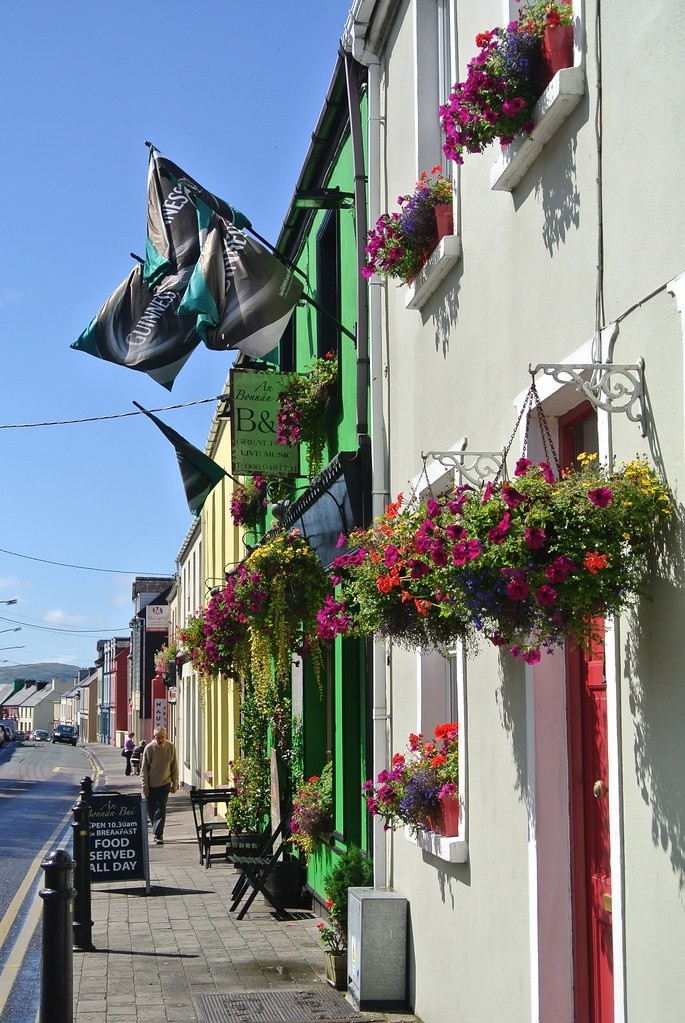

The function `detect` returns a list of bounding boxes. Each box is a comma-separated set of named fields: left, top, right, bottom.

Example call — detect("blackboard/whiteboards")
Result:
left=86, top=794, right=150, bottom=883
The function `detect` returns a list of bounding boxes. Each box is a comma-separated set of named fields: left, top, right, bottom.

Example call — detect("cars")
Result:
left=0, top=719, right=51, bottom=749
left=52, top=724, right=77, bottom=747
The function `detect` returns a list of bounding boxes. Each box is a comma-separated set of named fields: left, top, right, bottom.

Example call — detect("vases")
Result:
left=409, top=793, right=459, bottom=837
left=530, top=22, right=573, bottom=101
left=403, top=202, right=453, bottom=286
left=323, top=950, right=347, bottom=990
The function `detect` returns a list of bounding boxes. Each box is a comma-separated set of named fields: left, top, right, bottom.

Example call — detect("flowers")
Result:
left=278, top=346, right=338, bottom=446
left=153, top=450, right=674, bottom=866
left=438, top=0, right=573, bottom=165
left=361, top=163, right=458, bottom=289
left=316, top=899, right=348, bottom=955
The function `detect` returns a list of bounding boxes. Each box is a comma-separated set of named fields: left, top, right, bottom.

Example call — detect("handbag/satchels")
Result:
left=122, top=749, right=126, bottom=757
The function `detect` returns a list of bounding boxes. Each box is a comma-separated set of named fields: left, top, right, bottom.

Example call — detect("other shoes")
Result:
left=153, top=837, right=157, bottom=841
left=156, top=838, right=164, bottom=844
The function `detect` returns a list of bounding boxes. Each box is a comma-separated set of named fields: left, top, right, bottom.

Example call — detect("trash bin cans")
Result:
left=15, top=730, right=24, bottom=741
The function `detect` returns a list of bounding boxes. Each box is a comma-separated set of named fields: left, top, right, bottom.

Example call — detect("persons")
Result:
left=124, top=732, right=135, bottom=776
left=132, top=740, right=147, bottom=776
left=140, top=727, right=179, bottom=846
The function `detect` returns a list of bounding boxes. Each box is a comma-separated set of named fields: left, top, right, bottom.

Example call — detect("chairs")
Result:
left=226, top=802, right=299, bottom=920
left=189, top=784, right=237, bottom=868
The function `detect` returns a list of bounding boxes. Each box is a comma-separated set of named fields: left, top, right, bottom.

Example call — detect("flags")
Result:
left=142, top=144, right=304, bottom=359
left=70, top=261, right=203, bottom=392
left=150, top=412, right=225, bottom=517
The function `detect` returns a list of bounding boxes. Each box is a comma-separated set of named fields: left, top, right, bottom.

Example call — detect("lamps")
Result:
left=216, top=409, right=232, bottom=422
left=293, top=186, right=356, bottom=209
